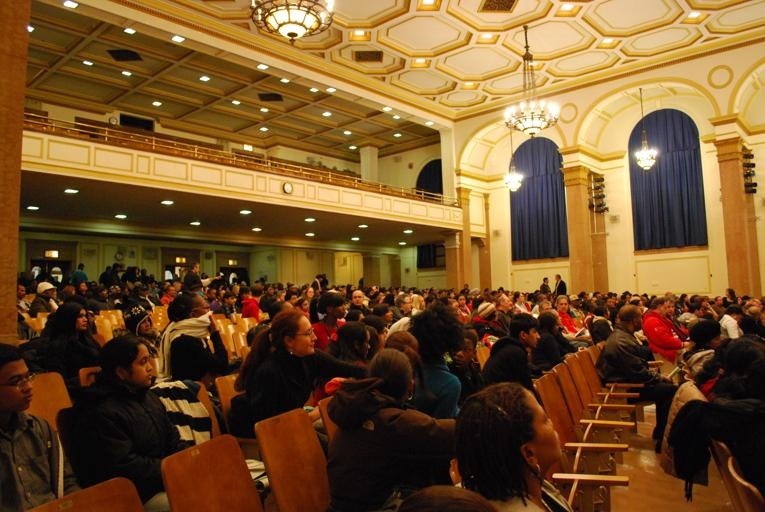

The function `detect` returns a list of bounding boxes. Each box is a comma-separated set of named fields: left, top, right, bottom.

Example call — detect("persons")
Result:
left=1, top=261, right=764, bottom=510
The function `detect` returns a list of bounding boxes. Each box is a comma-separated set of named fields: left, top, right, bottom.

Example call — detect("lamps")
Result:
left=504, top=25, right=561, bottom=138
left=634, top=88, right=658, bottom=172
left=249, top=0, right=334, bottom=48
left=504, top=130, right=524, bottom=192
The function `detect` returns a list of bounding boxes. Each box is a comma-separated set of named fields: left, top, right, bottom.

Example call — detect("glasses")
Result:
left=0, top=373, right=36, bottom=391
left=295, top=328, right=315, bottom=337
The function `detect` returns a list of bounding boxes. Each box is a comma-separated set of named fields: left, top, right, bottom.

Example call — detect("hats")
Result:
left=36, top=282, right=58, bottom=295
left=477, top=302, right=497, bottom=320
left=123, top=303, right=152, bottom=337
left=569, top=294, right=582, bottom=302
